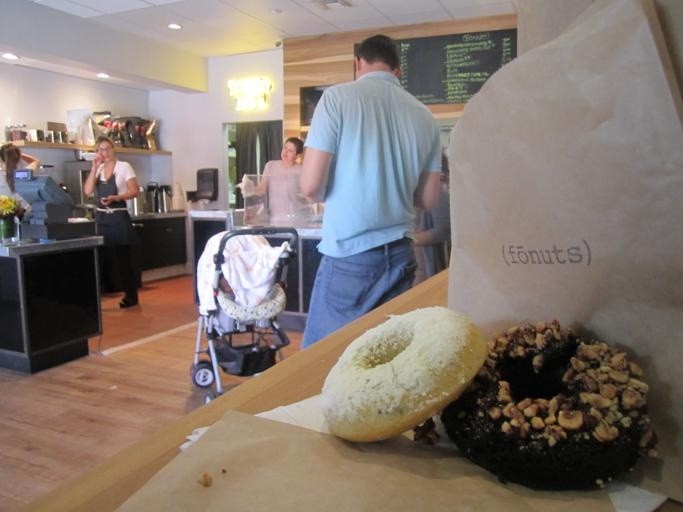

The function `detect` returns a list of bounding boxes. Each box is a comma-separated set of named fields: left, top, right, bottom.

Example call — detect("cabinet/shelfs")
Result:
left=130, top=217, right=186, bottom=271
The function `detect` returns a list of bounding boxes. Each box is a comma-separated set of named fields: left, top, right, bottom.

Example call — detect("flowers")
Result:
left=0, top=195, right=20, bottom=238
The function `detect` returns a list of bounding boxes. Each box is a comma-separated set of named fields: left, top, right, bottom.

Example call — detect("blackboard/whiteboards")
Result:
left=354, top=27, right=517, bottom=106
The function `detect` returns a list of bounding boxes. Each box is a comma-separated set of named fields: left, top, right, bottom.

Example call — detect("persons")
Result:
left=83, top=135, right=140, bottom=307
left=410, top=149, right=450, bottom=290
left=241, top=136, right=304, bottom=222
left=295, top=32, right=444, bottom=351
left=0, top=142, right=40, bottom=195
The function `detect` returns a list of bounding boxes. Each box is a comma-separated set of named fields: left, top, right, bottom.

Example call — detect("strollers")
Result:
left=191, top=223, right=299, bottom=405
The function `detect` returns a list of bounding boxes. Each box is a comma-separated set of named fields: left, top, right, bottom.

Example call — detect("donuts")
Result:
left=321, top=306, right=486, bottom=444
left=440, top=323, right=658, bottom=493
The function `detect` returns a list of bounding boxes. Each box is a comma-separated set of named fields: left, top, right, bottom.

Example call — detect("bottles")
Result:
left=145, top=180, right=174, bottom=213
left=6, top=120, right=28, bottom=142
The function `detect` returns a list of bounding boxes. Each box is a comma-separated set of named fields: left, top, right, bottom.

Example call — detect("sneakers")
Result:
left=120, top=297, right=138, bottom=307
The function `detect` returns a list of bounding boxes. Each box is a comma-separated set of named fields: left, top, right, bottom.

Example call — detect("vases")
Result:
left=0, top=215, right=19, bottom=247
